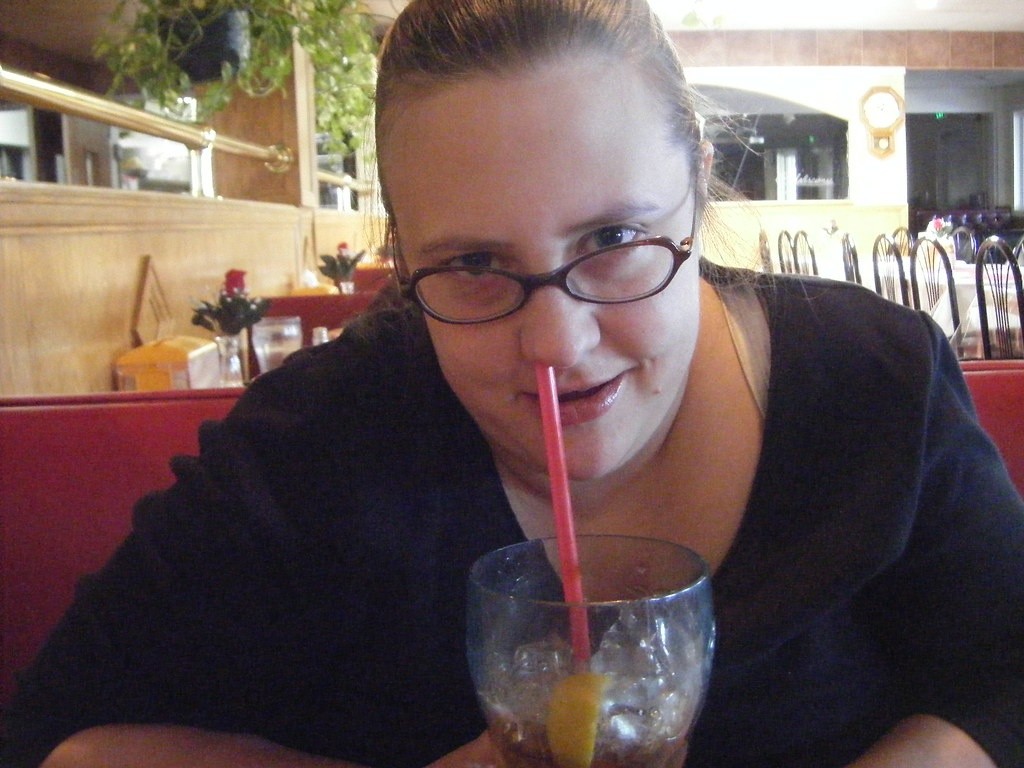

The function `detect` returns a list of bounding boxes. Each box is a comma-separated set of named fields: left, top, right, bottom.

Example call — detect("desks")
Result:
left=775, top=249, right=1024, bottom=360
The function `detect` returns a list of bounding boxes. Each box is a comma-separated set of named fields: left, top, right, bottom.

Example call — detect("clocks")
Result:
left=860, top=86, right=906, bottom=158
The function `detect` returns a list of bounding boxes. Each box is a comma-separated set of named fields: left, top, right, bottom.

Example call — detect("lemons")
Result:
left=547, top=672, right=610, bottom=768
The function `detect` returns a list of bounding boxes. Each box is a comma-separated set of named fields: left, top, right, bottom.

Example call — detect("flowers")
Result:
left=191, top=270, right=271, bottom=334
left=318, top=242, right=367, bottom=293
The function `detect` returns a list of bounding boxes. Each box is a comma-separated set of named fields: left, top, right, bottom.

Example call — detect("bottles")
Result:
left=312, top=326, right=328, bottom=346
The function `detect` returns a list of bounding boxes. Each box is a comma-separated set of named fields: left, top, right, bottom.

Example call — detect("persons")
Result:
left=0, top=0, right=1023, bottom=768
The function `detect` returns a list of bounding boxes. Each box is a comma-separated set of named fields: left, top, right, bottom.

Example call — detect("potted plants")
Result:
left=90, top=0, right=378, bottom=156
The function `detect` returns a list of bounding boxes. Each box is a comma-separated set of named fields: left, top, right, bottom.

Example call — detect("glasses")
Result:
left=391, top=113, right=707, bottom=326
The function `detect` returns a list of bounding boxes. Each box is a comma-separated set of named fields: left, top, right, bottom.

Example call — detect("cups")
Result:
left=464, top=535, right=717, bottom=767
left=252, top=315, right=302, bottom=373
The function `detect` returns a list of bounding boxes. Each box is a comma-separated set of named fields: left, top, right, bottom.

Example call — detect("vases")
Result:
left=214, top=338, right=242, bottom=389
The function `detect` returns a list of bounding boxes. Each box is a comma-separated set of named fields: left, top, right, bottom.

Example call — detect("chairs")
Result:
left=778, top=230, right=819, bottom=276
left=975, top=237, right=1024, bottom=361
left=759, top=232, right=773, bottom=272
left=892, top=226, right=915, bottom=257
left=911, top=237, right=964, bottom=355
left=871, top=234, right=906, bottom=306
left=842, top=233, right=862, bottom=285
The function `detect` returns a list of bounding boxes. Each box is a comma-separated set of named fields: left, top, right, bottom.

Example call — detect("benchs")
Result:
left=916, top=208, right=1011, bottom=238
left=354, top=267, right=400, bottom=292
left=248, top=295, right=376, bottom=381
left=960, top=363, right=1024, bottom=495
left=1, top=388, right=253, bottom=698
